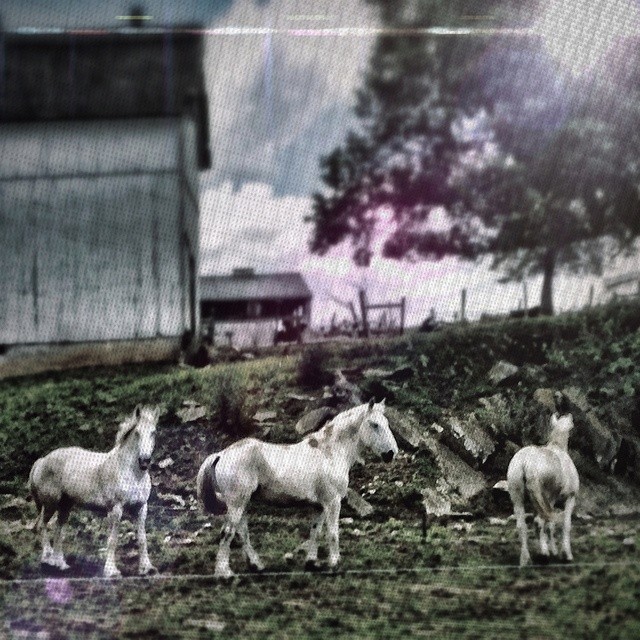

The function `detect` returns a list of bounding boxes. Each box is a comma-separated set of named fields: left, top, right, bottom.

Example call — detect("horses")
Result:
left=506, top=412, right=579, bottom=572
left=196, top=392, right=398, bottom=576
left=25, top=400, right=160, bottom=580
